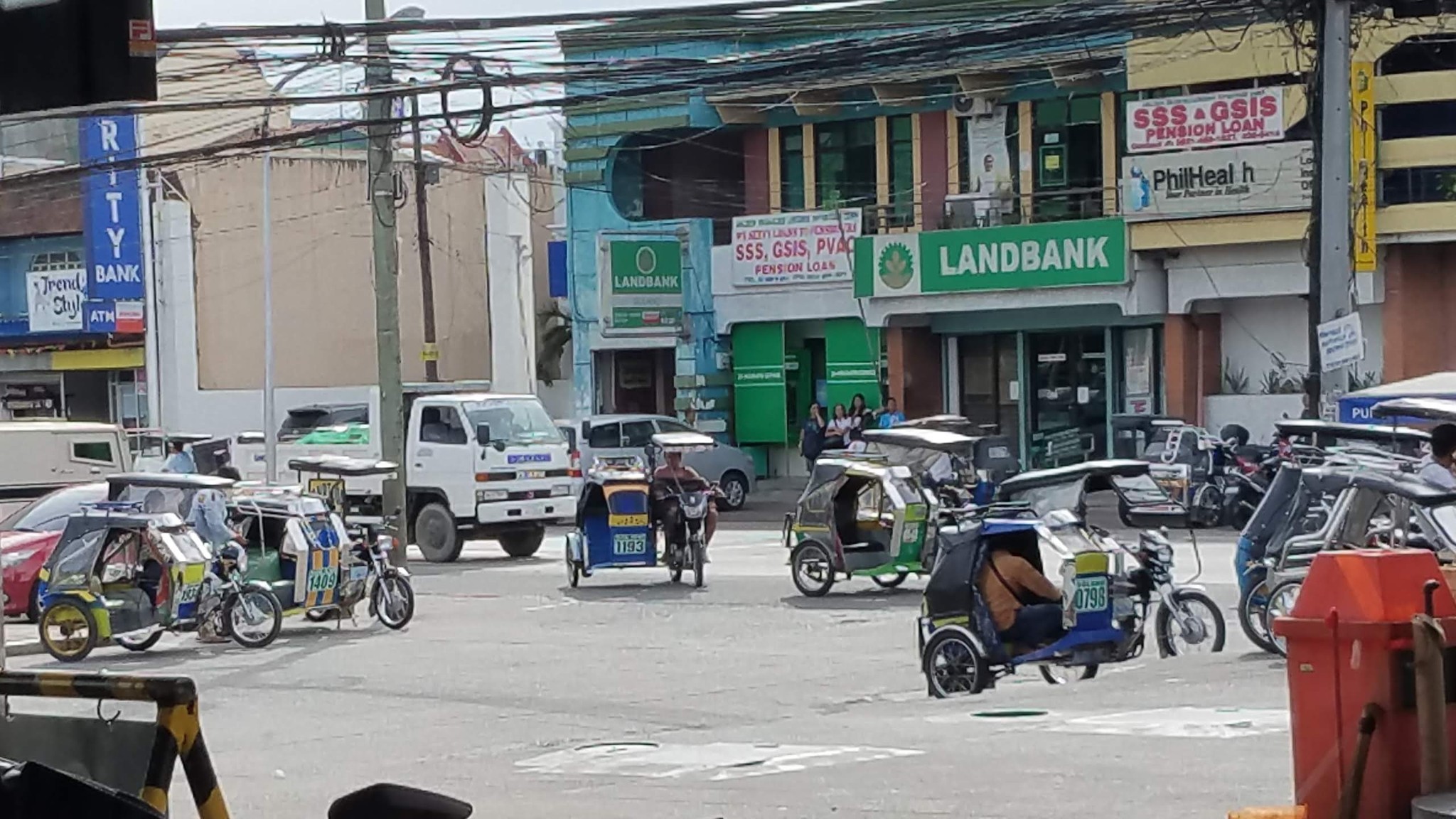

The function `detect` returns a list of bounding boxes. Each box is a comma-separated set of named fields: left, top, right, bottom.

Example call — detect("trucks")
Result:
left=228, top=378, right=578, bottom=563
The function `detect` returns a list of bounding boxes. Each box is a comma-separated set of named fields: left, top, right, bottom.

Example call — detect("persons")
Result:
left=976, top=155, right=1000, bottom=192
left=1418, top=423, right=1456, bottom=555
left=144, top=470, right=249, bottom=644
left=654, top=450, right=717, bottom=562
left=974, top=531, right=1071, bottom=644
left=797, top=394, right=903, bottom=477
left=161, top=441, right=194, bottom=474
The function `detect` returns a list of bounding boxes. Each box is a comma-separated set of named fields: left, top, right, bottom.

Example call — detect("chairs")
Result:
left=421, top=407, right=451, bottom=443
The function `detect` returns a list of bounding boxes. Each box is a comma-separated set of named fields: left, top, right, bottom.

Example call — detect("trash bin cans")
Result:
left=1273, top=548, right=1456, bottom=819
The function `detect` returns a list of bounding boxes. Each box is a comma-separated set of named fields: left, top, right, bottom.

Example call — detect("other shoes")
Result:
left=701, top=552, right=711, bottom=562
left=662, top=554, right=666, bottom=561
left=202, top=636, right=232, bottom=642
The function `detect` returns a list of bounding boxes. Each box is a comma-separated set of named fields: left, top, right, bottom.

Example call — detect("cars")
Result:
left=278, top=401, right=369, bottom=443
left=555, top=415, right=758, bottom=510
left=0, top=482, right=106, bottom=624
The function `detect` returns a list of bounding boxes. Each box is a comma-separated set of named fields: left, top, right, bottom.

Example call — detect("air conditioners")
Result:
left=952, top=84, right=993, bottom=119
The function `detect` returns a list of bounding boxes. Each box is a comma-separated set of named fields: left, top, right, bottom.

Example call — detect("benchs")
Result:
left=837, top=522, right=885, bottom=552
left=247, top=546, right=282, bottom=585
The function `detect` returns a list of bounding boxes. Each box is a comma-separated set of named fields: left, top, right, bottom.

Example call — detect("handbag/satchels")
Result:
left=849, top=427, right=864, bottom=440
left=824, top=436, right=844, bottom=448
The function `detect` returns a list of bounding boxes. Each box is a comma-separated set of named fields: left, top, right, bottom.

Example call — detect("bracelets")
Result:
left=798, top=438, right=804, bottom=441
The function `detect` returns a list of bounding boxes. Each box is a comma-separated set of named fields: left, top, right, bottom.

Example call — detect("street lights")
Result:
left=262, top=5, right=426, bottom=482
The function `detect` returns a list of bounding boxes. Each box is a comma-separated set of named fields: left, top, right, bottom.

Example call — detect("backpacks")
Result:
left=803, top=427, right=821, bottom=460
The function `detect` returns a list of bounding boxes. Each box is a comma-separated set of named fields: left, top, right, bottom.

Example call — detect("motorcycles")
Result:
left=564, top=430, right=728, bottom=588
left=37, top=472, right=285, bottom=663
left=915, top=457, right=1226, bottom=699
left=784, top=428, right=986, bottom=598
left=227, top=454, right=415, bottom=629
left=1119, top=396, right=1455, bottom=660
